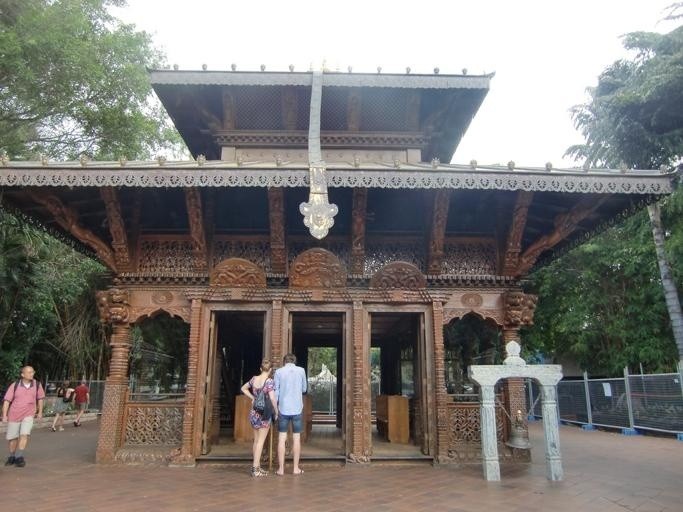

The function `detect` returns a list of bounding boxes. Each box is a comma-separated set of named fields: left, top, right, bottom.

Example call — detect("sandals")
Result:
left=251, top=467, right=270, bottom=476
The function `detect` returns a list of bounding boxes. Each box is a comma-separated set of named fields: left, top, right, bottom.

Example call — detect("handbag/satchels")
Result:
left=253, top=377, right=274, bottom=420
left=63, top=398, right=69, bottom=402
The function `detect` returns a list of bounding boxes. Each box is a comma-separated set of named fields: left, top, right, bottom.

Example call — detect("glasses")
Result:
left=27, top=371, right=35, bottom=375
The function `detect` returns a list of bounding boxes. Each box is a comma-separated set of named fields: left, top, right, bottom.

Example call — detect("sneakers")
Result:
left=73, top=421, right=81, bottom=426
left=4, top=456, right=25, bottom=466
left=50, top=426, right=65, bottom=431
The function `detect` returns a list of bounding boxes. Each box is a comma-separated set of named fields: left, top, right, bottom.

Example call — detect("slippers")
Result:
left=293, top=470, right=304, bottom=475
left=274, top=471, right=282, bottom=476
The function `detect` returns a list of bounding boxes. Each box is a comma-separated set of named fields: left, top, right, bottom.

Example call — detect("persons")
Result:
left=71, top=380, right=89, bottom=427
left=49, top=379, right=72, bottom=432
left=1, top=365, right=46, bottom=468
left=272, top=352, right=308, bottom=476
left=240, top=359, right=278, bottom=477
